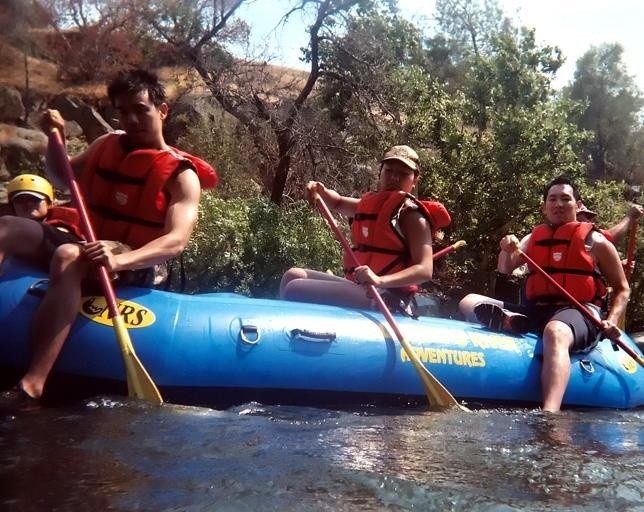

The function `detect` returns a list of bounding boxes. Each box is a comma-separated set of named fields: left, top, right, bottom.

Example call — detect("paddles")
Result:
left=48, top=122, right=164, bottom=409
left=307, top=181, right=461, bottom=413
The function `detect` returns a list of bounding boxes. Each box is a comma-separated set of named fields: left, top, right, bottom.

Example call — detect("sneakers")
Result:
left=473, top=302, right=531, bottom=335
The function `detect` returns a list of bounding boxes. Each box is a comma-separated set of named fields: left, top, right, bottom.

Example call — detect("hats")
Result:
left=576, top=203, right=598, bottom=220
left=380, top=144, right=421, bottom=176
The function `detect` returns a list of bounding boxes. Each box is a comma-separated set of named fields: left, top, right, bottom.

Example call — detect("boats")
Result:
left=0, top=254, right=644, bottom=411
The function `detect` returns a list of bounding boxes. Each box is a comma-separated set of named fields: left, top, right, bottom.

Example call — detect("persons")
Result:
left=456, top=174, right=632, bottom=415
left=277, top=143, right=451, bottom=309
left=518, top=418, right=604, bottom=508
left=574, top=200, right=644, bottom=306
left=2, top=172, right=83, bottom=266
left=1, top=61, right=218, bottom=410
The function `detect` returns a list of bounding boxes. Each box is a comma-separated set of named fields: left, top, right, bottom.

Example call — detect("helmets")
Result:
left=7, top=173, right=53, bottom=208
